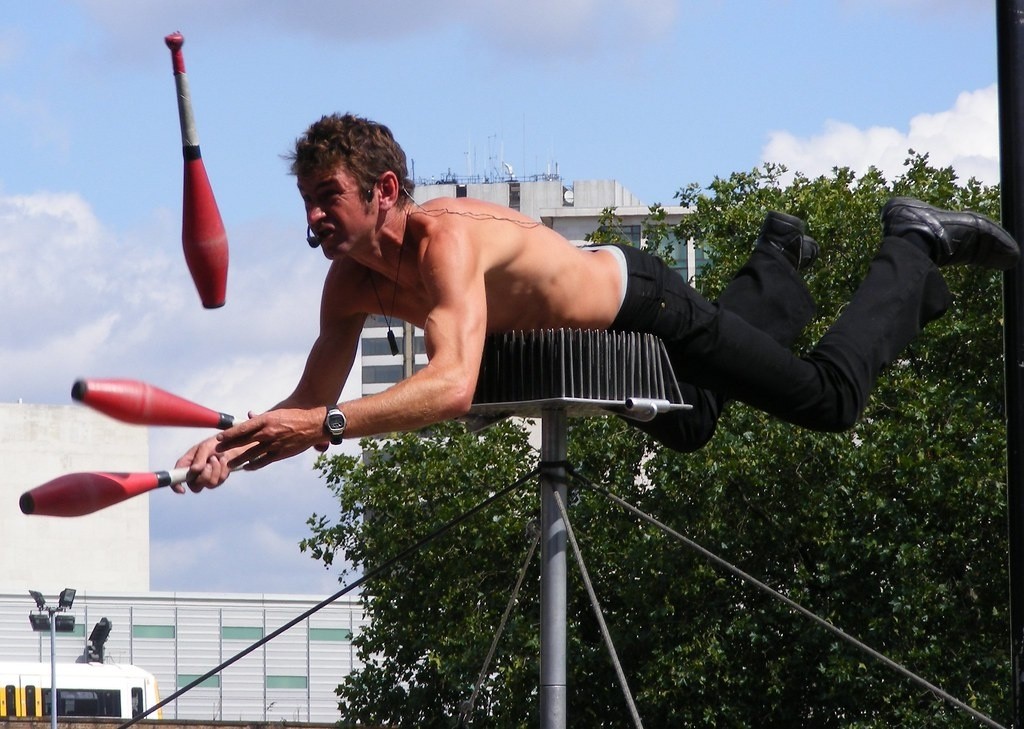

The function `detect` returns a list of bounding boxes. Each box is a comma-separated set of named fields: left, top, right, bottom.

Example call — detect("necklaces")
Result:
left=368, top=199, right=414, bottom=356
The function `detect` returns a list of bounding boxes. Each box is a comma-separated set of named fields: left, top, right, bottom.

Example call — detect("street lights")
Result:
left=28, top=588, right=76, bottom=728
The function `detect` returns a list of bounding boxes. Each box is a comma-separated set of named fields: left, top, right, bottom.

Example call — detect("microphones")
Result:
left=306, top=225, right=321, bottom=249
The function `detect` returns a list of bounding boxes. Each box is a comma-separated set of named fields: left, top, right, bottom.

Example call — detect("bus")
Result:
left=0, top=662, right=161, bottom=720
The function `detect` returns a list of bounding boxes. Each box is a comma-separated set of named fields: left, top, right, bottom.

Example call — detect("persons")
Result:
left=169, top=114, right=1020, bottom=495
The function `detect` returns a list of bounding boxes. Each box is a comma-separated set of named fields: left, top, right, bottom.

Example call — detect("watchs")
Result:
left=325, top=404, right=347, bottom=445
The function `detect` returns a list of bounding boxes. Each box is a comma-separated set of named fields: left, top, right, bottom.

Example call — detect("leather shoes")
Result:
left=879, top=196, right=1022, bottom=273
left=758, top=210, right=818, bottom=273
